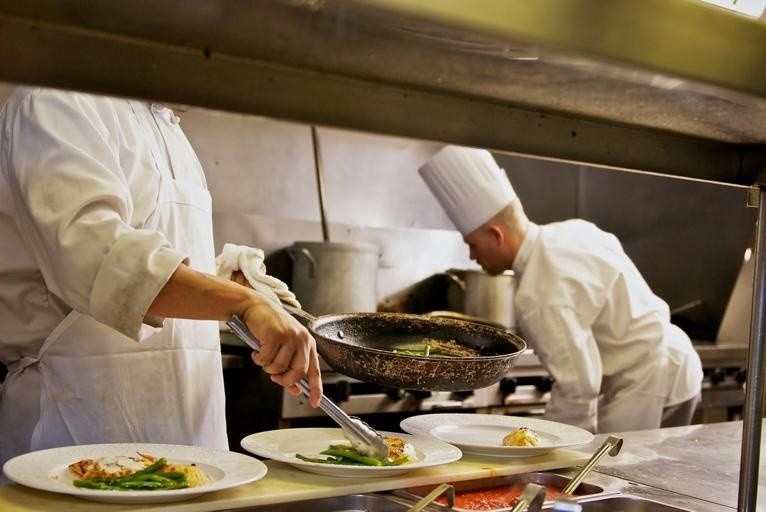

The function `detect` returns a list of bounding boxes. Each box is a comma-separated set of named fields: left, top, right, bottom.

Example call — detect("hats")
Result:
left=419, top=145, right=518, bottom=237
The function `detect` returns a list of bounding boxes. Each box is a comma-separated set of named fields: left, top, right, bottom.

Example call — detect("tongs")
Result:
left=226, top=314, right=389, bottom=462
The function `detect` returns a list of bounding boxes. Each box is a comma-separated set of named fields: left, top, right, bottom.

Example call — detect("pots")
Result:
left=268, top=295, right=528, bottom=392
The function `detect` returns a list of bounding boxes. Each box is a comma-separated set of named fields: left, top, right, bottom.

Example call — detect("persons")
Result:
left=459, top=192, right=705, bottom=433
left=1, top=84, right=329, bottom=489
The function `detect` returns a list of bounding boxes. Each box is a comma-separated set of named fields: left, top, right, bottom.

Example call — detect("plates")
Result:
left=241, top=427, right=463, bottom=480
left=399, top=412, right=596, bottom=458
left=1, top=442, right=267, bottom=504
